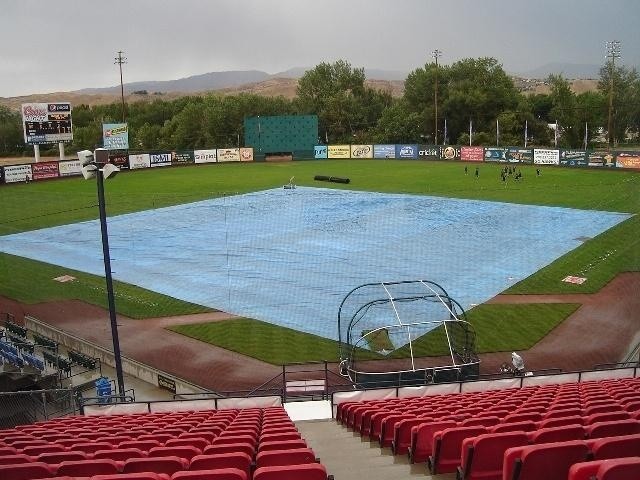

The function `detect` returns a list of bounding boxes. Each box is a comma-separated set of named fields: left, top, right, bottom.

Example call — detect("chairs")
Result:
left=335, top=375, right=640, bottom=479
left=1, top=320, right=335, bottom=480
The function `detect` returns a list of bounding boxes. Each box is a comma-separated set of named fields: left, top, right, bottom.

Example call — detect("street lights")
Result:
left=76, top=147, right=129, bottom=403
left=113, top=50, right=128, bottom=124
left=431, top=49, right=443, bottom=146
left=605, top=40, right=622, bottom=152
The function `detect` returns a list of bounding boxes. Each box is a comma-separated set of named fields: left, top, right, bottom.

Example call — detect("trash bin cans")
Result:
left=94, top=377, right=112, bottom=404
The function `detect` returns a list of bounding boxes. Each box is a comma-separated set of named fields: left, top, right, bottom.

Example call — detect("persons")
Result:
left=536, top=169, right=542, bottom=178
left=473, top=168, right=480, bottom=184
left=464, top=165, right=469, bottom=176
left=498, top=164, right=524, bottom=190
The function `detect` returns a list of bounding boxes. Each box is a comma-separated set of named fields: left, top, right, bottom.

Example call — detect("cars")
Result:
left=510, top=76, right=551, bottom=93
